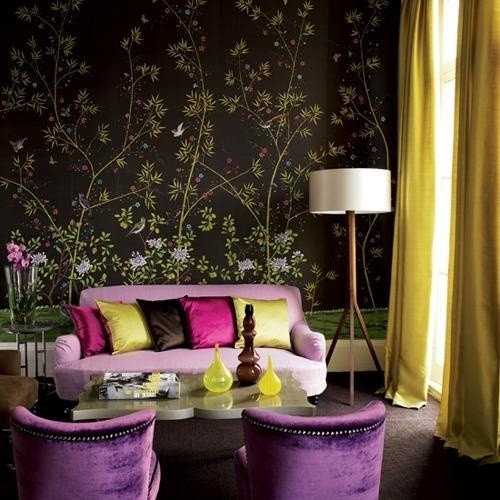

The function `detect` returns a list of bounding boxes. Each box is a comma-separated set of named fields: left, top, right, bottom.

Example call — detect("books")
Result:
left=97, top=371, right=181, bottom=401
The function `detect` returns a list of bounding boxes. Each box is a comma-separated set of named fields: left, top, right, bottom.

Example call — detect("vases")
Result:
left=5, top=264, right=38, bottom=331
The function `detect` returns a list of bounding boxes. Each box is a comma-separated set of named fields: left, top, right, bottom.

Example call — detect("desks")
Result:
left=0, top=320, right=56, bottom=395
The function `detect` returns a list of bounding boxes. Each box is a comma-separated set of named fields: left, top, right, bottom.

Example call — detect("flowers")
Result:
left=5, top=241, right=36, bottom=325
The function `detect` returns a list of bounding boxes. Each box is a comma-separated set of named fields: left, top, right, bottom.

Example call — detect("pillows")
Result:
left=60, top=295, right=292, bottom=359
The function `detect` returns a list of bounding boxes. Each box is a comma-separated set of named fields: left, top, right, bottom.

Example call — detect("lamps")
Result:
left=310, top=169, right=393, bottom=406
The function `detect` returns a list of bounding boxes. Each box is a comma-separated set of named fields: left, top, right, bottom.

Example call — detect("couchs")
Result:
left=54, top=284, right=327, bottom=405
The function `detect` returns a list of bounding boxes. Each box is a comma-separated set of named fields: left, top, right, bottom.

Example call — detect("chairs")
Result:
left=234, top=400, right=386, bottom=500
left=10, top=405, right=161, bottom=500
left=0, top=350, right=39, bottom=431
